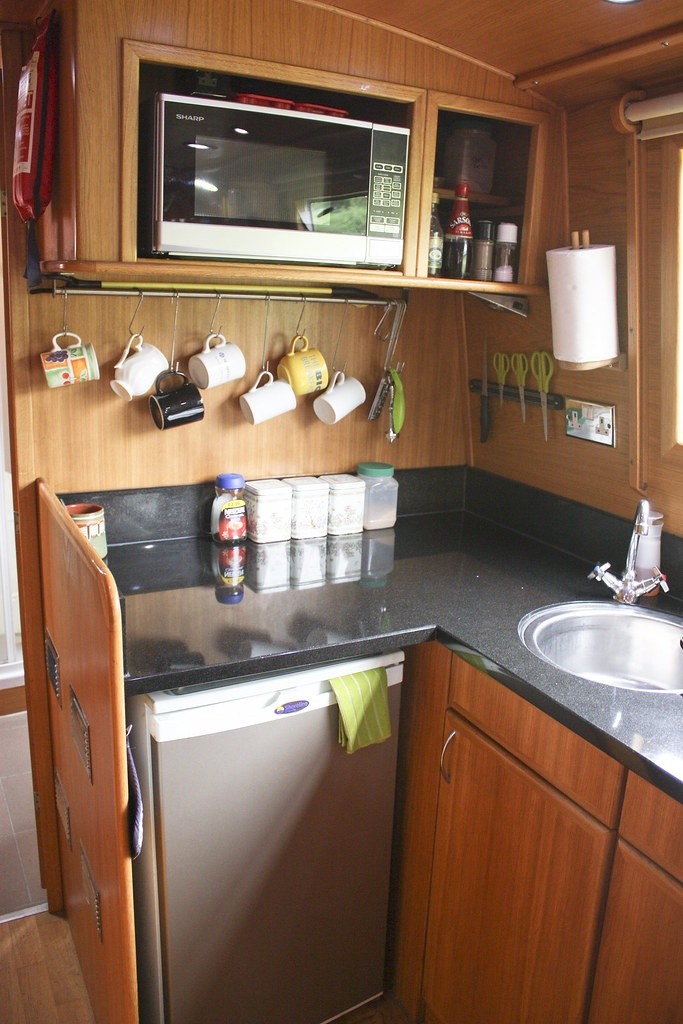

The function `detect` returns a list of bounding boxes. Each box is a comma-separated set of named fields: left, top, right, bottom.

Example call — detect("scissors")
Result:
left=531, top=351, right=553, bottom=441
left=510, top=353, right=529, bottom=424
left=492, top=352, right=510, bottom=408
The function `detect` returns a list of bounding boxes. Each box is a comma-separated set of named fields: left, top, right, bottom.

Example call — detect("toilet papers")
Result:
left=545, top=243, right=619, bottom=365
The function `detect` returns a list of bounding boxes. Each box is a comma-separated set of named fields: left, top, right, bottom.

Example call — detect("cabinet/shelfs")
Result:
left=386, top=644, right=682, bottom=1024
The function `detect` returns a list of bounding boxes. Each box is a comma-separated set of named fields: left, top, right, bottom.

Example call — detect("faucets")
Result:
left=616, top=500, right=650, bottom=601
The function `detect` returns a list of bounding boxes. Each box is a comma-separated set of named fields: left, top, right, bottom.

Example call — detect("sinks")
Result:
left=516, top=601, right=683, bottom=695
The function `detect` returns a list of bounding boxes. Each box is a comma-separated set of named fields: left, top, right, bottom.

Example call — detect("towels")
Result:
left=329, top=667, right=393, bottom=754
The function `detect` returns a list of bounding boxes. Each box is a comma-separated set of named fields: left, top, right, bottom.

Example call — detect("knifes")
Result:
left=481, top=339, right=490, bottom=442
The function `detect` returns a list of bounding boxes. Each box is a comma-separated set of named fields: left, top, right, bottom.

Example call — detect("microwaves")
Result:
left=138, top=90, right=411, bottom=270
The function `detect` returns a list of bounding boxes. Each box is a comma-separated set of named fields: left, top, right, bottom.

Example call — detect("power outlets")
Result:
left=563, top=398, right=617, bottom=447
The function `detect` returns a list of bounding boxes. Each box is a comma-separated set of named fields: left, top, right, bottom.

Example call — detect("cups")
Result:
left=189, top=333, right=246, bottom=389
left=148, top=370, right=205, bottom=431
left=109, top=334, right=170, bottom=401
left=239, top=371, right=297, bottom=425
left=40, top=332, right=100, bottom=388
left=313, top=371, right=366, bottom=425
left=277, top=336, right=329, bottom=396
left=66, top=503, right=108, bottom=560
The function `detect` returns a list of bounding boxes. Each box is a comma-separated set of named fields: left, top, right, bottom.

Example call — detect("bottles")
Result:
left=212, top=543, right=247, bottom=605
left=443, top=120, right=497, bottom=194
left=355, top=461, right=399, bottom=530
left=428, top=185, right=473, bottom=280
left=210, top=473, right=248, bottom=544
left=357, top=528, right=396, bottom=588
left=470, top=220, right=518, bottom=281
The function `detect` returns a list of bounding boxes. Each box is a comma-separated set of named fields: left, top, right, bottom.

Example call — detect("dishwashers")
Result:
left=126, top=651, right=405, bottom=1024
